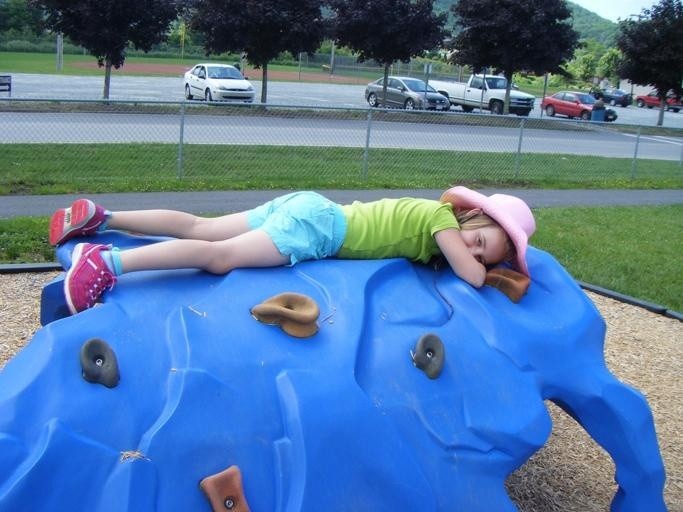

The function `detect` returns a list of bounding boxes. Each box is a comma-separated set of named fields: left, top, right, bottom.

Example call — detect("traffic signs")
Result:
left=0, top=76, right=11, bottom=92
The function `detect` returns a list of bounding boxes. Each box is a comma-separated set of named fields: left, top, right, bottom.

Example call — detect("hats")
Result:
left=441, top=186, right=535, bottom=278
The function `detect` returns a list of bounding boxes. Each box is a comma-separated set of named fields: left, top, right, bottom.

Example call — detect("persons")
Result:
left=46, top=181, right=538, bottom=318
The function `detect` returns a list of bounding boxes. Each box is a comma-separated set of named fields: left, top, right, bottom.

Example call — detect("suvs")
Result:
left=636, top=87, right=682, bottom=113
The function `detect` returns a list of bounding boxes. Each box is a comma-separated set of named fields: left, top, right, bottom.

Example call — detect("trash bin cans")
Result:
left=592, top=106, right=605, bottom=121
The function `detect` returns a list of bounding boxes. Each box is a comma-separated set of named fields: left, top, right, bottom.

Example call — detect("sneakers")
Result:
left=64, top=243, right=115, bottom=315
left=50, top=199, right=108, bottom=246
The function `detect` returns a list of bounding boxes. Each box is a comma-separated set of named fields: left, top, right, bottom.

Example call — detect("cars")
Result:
left=183, top=64, right=255, bottom=105
left=594, top=89, right=632, bottom=108
left=364, top=76, right=450, bottom=114
left=541, top=92, right=616, bottom=122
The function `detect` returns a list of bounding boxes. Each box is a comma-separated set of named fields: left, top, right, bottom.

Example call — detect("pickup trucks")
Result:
left=427, top=72, right=534, bottom=118
